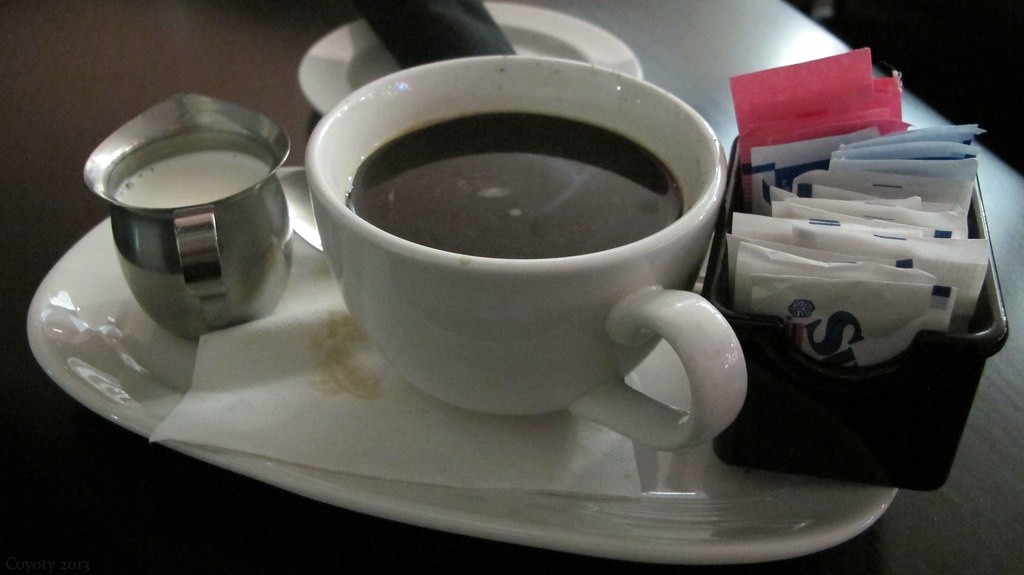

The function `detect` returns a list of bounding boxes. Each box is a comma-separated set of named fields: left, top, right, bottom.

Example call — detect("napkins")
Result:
left=147, top=218, right=646, bottom=502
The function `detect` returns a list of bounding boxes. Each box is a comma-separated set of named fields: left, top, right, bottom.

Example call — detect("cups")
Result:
left=307, top=56, right=749, bottom=452
left=82, top=92, right=291, bottom=339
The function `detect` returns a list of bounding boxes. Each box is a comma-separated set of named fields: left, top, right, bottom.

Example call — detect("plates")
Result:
left=297, top=0, right=646, bottom=118
left=27, top=163, right=899, bottom=561
